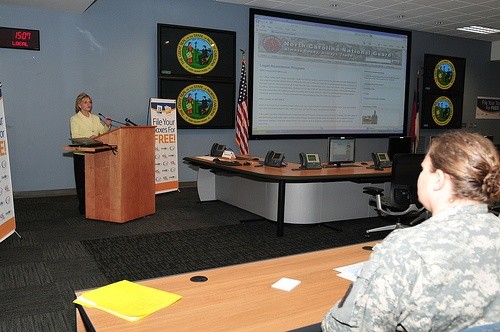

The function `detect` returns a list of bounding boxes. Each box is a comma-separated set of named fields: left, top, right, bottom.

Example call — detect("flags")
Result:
left=409, top=78, right=420, bottom=154
left=235, top=57, right=250, bottom=155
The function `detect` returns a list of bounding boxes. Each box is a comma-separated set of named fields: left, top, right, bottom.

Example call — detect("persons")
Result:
left=320, top=131, right=500, bottom=332
left=71, top=94, right=111, bottom=215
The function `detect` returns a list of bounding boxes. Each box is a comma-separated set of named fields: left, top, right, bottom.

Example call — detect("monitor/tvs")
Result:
left=387, top=136, right=412, bottom=162
left=328, top=137, right=356, bottom=167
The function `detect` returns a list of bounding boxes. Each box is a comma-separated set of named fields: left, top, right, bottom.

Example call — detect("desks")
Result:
left=74, top=239, right=381, bottom=332
left=183, top=155, right=392, bottom=236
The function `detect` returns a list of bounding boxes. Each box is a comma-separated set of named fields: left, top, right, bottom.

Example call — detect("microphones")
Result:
left=125, top=118, right=138, bottom=125
left=98, top=112, right=130, bottom=125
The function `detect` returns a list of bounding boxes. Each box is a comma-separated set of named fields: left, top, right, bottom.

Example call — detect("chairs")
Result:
left=362, top=153, right=431, bottom=234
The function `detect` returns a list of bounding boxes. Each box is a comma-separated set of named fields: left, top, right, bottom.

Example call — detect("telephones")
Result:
left=264, top=151, right=285, bottom=167
left=210, top=143, right=226, bottom=157
left=299, top=153, right=321, bottom=169
left=371, top=151, right=391, bottom=168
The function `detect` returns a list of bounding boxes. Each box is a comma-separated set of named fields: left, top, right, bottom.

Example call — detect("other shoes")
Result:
left=79, top=209, right=85, bottom=216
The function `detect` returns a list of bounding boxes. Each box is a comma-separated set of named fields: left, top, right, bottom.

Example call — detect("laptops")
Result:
left=68, top=137, right=103, bottom=147
